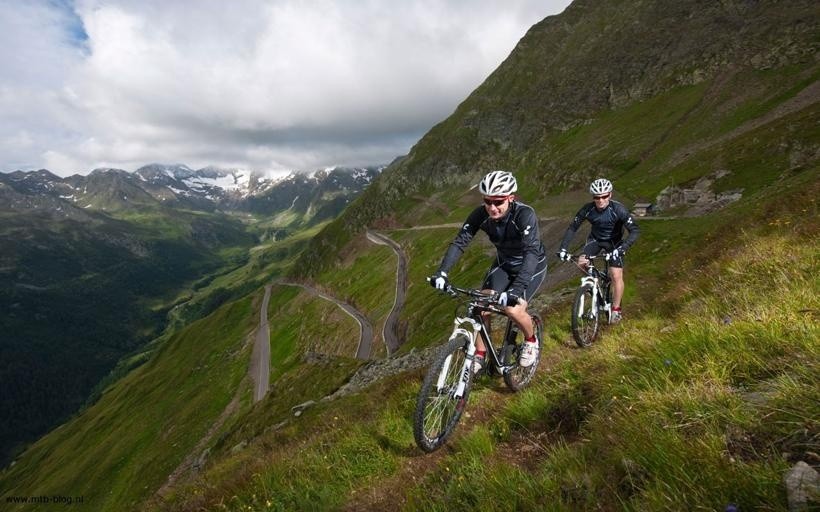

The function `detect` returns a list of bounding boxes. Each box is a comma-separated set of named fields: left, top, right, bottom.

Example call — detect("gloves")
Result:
left=558, top=248, right=568, bottom=261
left=611, top=247, right=623, bottom=260
left=431, top=270, right=450, bottom=291
left=497, top=290, right=518, bottom=307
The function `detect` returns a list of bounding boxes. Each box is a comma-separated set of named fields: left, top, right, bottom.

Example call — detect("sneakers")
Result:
left=472, top=356, right=484, bottom=373
left=520, top=334, right=540, bottom=367
left=610, top=310, right=622, bottom=324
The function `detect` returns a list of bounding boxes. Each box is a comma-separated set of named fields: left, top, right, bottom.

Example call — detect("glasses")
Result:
left=593, top=195, right=610, bottom=200
left=482, top=196, right=506, bottom=206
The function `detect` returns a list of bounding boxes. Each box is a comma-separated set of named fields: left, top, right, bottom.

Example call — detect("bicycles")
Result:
left=414, top=277, right=543, bottom=453
left=555, top=251, right=626, bottom=347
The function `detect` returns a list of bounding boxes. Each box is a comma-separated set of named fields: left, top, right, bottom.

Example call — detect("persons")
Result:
left=432, top=171, right=548, bottom=374
left=560, top=179, right=640, bottom=325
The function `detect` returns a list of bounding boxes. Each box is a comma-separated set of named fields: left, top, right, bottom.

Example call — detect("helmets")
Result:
left=590, top=179, right=613, bottom=198
left=479, top=170, right=518, bottom=199
